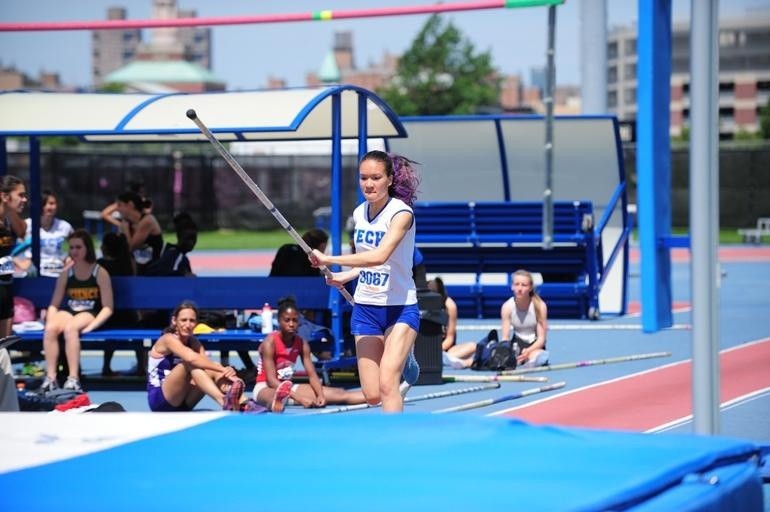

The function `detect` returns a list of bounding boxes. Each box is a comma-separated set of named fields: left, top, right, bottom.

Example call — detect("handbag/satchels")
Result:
left=471, top=330, right=515, bottom=370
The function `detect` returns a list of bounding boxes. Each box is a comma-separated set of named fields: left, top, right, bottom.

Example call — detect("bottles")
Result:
left=261, top=302, right=273, bottom=335
left=512, top=342, right=520, bottom=367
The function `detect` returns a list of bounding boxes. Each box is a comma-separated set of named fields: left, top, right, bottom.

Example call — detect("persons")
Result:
left=309, top=151, right=422, bottom=415
left=422, top=276, right=477, bottom=372
left=250, top=295, right=411, bottom=414
left=448, top=271, right=550, bottom=370
left=270, top=229, right=328, bottom=277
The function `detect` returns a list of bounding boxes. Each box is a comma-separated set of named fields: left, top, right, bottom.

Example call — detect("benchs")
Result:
left=10, top=273, right=333, bottom=377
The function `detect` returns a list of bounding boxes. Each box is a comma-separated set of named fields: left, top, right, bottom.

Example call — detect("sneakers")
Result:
left=40, top=376, right=84, bottom=392
left=224, top=380, right=270, bottom=414
left=403, top=340, right=419, bottom=385
left=271, top=380, right=294, bottom=413
